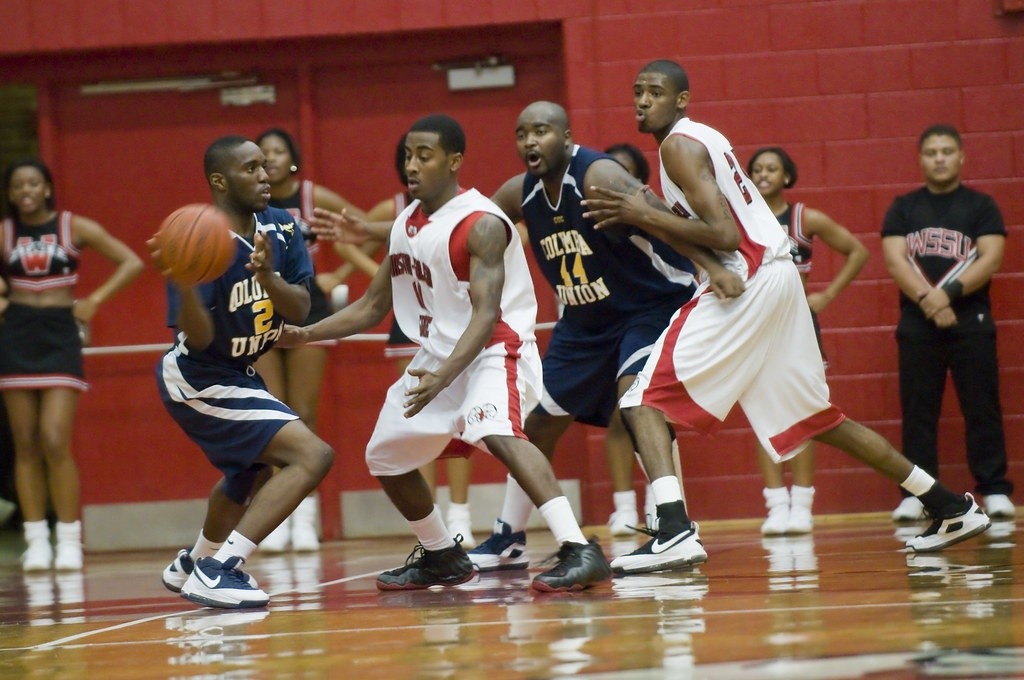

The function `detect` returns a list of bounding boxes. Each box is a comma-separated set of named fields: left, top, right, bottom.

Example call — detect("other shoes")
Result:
left=983, top=494, right=1014, bottom=517
left=891, top=497, right=929, bottom=522
left=52, top=522, right=84, bottom=570
left=23, top=518, right=51, bottom=570
left=608, top=491, right=638, bottom=535
left=446, top=516, right=477, bottom=549
left=760, top=485, right=816, bottom=534
left=263, top=498, right=319, bottom=551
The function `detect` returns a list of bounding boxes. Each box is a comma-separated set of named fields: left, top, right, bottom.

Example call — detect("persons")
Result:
left=594, top=141, right=692, bottom=536
left=0, top=153, right=143, bottom=572
left=878, top=124, right=1019, bottom=523
left=577, top=60, right=993, bottom=573
left=247, top=128, right=379, bottom=556
left=271, top=114, right=612, bottom=591
left=146, top=134, right=337, bottom=610
left=303, top=102, right=747, bottom=571
left=311, top=128, right=482, bottom=553
left=747, top=144, right=869, bottom=536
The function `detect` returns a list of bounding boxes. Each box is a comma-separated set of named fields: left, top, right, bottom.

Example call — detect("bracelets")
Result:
left=942, top=278, right=965, bottom=305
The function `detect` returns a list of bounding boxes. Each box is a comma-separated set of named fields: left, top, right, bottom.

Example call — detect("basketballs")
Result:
left=154, top=202, right=237, bottom=290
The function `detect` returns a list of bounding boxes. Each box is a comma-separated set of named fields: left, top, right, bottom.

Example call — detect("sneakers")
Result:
left=180, top=554, right=270, bottom=608
left=467, top=517, right=530, bottom=572
left=161, top=545, right=259, bottom=593
left=533, top=541, right=613, bottom=591
left=376, top=532, right=475, bottom=590
left=610, top=512, right=708, bottom=575
left=905, top=492, right=992, bottom=554
